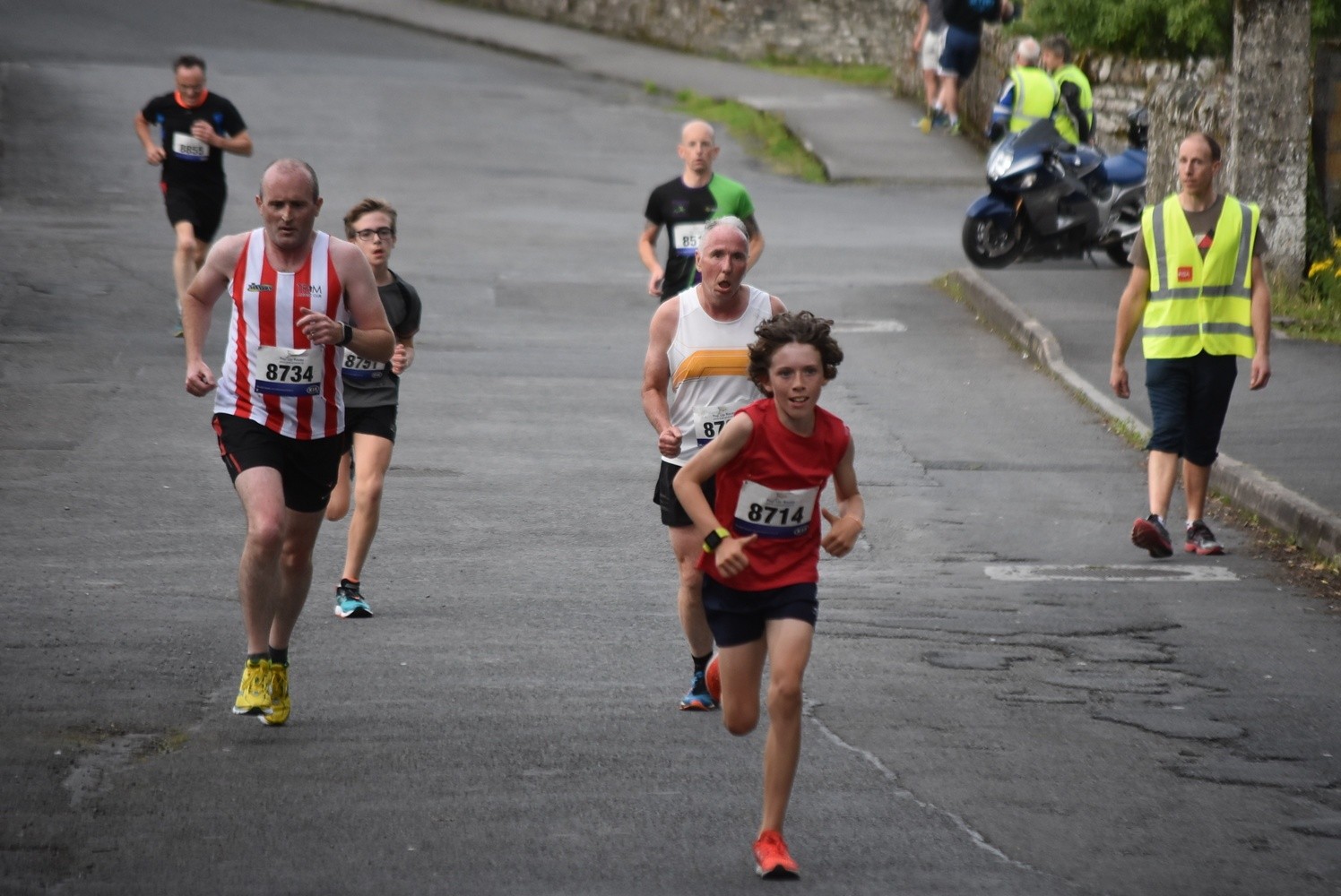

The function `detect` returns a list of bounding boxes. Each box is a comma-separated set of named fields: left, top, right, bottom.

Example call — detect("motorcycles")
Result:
left=960, top=116, right=1147, bottom=268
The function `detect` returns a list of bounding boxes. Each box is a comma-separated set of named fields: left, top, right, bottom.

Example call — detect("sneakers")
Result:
left=259, top=656, right=291, bottom=725
left=1132, top=514, right=1175, bottom=557
left=334, top=577, right=373, bottom=619
left=231, top=655, right=272, bottom=713
left=753, top=830, right=801, bottom=879
left=1183, top=520, right=1224, bottom=554
left=704, top=652, right=727, bottom=700
left=680, top=673, right=714, bottom=709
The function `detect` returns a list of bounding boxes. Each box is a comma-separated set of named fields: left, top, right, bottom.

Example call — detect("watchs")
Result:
left=702, top=526, right=730, bottom=554
left=333, top=321, right=352, bottom=347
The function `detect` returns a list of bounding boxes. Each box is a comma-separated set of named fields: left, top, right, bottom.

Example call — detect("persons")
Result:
left=638, top=119, right=765, bottom=307
left=179, top=155, right=396, bottom=727
left=671, top=309, right=865, bottom=882
left=134, top=54, right=253, bottom=341
left=1110, top=132, right=1272, bottom=559
left=641, top=217, right=789, bottom=713
left=910, top=0, right=1097, bottom=149
left=323, top=199, right=423, bottom=619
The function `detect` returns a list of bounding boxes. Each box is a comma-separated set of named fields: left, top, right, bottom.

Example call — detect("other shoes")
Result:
left=172, top=317, right=183, bottom=336
left=949, top=122, right=962, bottom=136
left=921, top=107, right=936, bottom=133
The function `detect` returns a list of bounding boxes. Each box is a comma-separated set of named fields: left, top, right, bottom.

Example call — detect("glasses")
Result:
left=354, top=227, right=395, bottom=242
